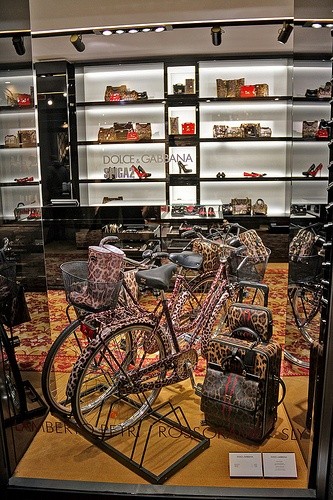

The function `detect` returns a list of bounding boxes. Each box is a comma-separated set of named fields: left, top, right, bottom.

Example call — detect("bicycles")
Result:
left=41, top=222, right=329, bottom=439
left=0, top=238, right=30, bottom=427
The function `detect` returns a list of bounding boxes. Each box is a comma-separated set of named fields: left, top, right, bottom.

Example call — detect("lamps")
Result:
left=70, top=34, right=85, bottom=52
left=12, top=36, right=25, bottom=56
left=47, top=95, right=54, bottom=105
left=211, top=27, right=222, bottom=46
left=277, top=20, right=294, bottom=45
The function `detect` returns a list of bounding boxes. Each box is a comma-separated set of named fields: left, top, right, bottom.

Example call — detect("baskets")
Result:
left=290, top=255, right=323, bottom=284
left=59, top=261, right=124, bottom=313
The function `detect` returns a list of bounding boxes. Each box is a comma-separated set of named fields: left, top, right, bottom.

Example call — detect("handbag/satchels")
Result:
left=291, top=203, right=307, bottom=216
left=215, top=78, right=269, bottom=98
left=300, top=119, right=333, bottom=140
left=88, top=244, right=125, bottom=300
left=98, top=121, right=152, bottom=143
left=317, top=87, right=331, bottom=99
left=193, top=230, right=268, bottom=277
left=17, top=94, right=32, bottom=106
left=213, top=123, right=272, bottom=138
left=228, top=302, right=273, bottom=342
left=223, top=198, right=267, bottom=215
left=288, top=229, right=315, bottom=256
left=14, top=202, right=41, bottom=221
left=226, top=229, right=268, bottom=276
left=305, top=88, right=319, bottom=97
left=105, top=85, right=148, bottom=101
left=173, top=84, right=186, bottom=93
left=5, top=130, right=37, bottom=147
left=181, top=122, right=195, bottom=134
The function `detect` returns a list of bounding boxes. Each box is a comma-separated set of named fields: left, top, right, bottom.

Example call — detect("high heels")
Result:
left=131, top=165, right=152, bottom=177
left=302, top=163, right=323, bottom=178
left=178, top=161, right=192, bottom=173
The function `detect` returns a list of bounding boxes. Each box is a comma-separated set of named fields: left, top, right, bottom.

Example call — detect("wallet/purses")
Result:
left=185, top=79, right=194, bottom=94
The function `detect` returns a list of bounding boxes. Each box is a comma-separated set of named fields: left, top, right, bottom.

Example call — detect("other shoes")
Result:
left=244, top=171, right=267, bottom=178
left=171, top=205, right=217, bottom=217
left=13, top=176, right=33, bottom=182
left=217, top=172, right=226, bottom=178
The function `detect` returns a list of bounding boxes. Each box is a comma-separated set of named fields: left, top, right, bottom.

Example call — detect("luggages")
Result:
left=199, top=331, right=282, bottom=444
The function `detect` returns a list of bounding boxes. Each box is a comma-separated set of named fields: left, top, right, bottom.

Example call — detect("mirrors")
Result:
left=282, top=0, right=333, bottom=471
left=1, top=0, right=58, bottom=476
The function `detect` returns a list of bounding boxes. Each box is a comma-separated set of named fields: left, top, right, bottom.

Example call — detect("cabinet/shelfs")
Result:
left=0, top=61, right=42, bottom=221
left=287, top=56, right=332, bottom=216
left=68, top=55, right=286, bottom=221
